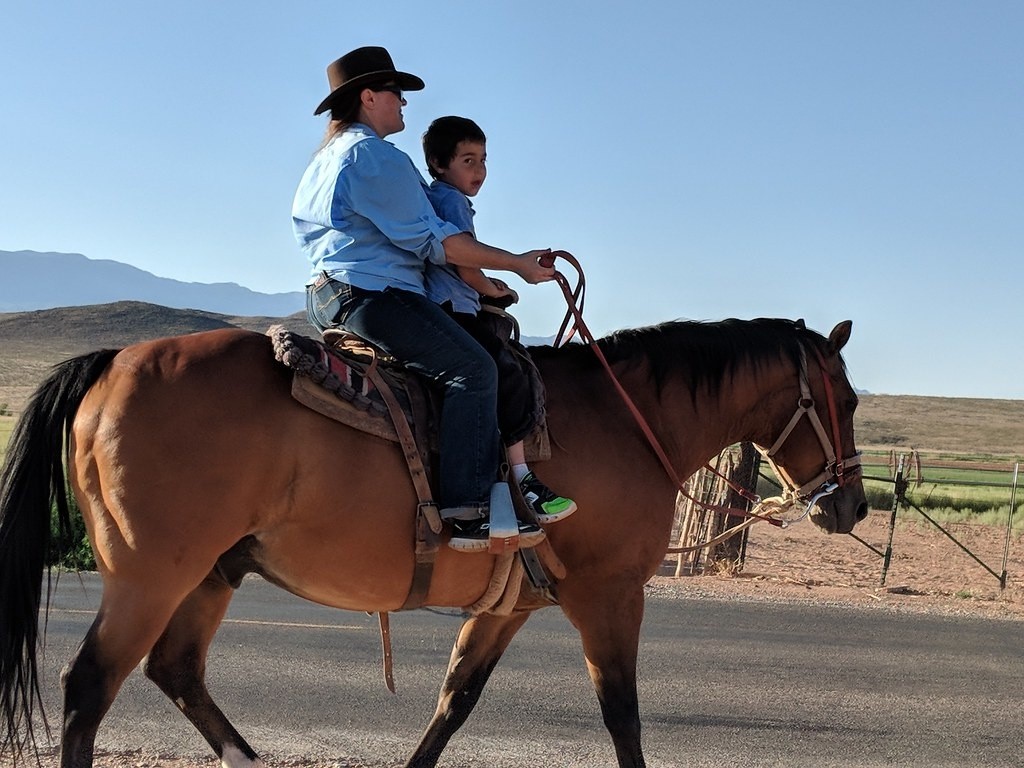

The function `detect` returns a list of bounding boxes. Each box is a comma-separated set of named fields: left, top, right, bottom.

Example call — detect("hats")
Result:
left=314, top=45, right=425, bottom=118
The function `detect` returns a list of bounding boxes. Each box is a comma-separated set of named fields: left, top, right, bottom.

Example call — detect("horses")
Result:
left=0, top=317, right=869, bottom=768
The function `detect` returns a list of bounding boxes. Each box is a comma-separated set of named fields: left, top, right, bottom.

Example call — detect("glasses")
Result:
left=365, top=83, right=404, bottom=102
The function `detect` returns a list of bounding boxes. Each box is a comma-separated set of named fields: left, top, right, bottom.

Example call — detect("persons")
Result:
left=291, top=46, right=547, bottom=553
left=422, top=116, right=578, bottom=524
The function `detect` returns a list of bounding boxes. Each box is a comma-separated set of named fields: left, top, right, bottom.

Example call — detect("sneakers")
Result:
left=447, top=512, right=547, bottom=553
left=520, top=469, right=578, bottom=524
